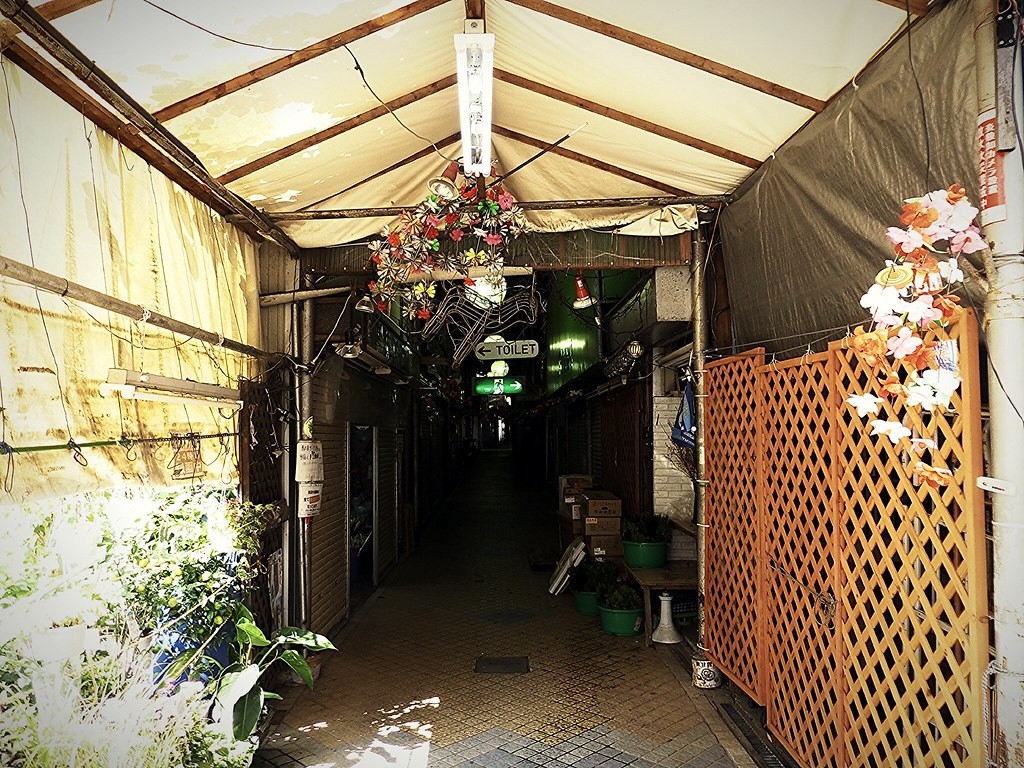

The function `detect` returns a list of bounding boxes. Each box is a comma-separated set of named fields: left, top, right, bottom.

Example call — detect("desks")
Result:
left=625, top=561, right=697, bottom=648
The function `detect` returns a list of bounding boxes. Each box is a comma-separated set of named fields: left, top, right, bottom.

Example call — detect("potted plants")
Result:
left=566, top=513, right=672, bottom=637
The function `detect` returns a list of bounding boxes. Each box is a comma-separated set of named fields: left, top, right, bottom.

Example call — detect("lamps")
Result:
left=341, top=331, right=359, bottom=359
left=427, top=161, right=460, bottom=201
left=453, top=19, right=495, bottom=179
left=573, top=276, right=598, bottom=308
left=355, top=281, right=375, bottom=313
left=97, top=304, right=244, bottom=412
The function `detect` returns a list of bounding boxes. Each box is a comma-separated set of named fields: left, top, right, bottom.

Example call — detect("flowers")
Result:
left=846, top=182, right=988, bottom=488
left=368, top=165, right=533, bottom=319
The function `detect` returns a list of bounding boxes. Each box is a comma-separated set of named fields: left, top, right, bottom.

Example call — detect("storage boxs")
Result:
left=556, top=473, right=623, bottom=563
